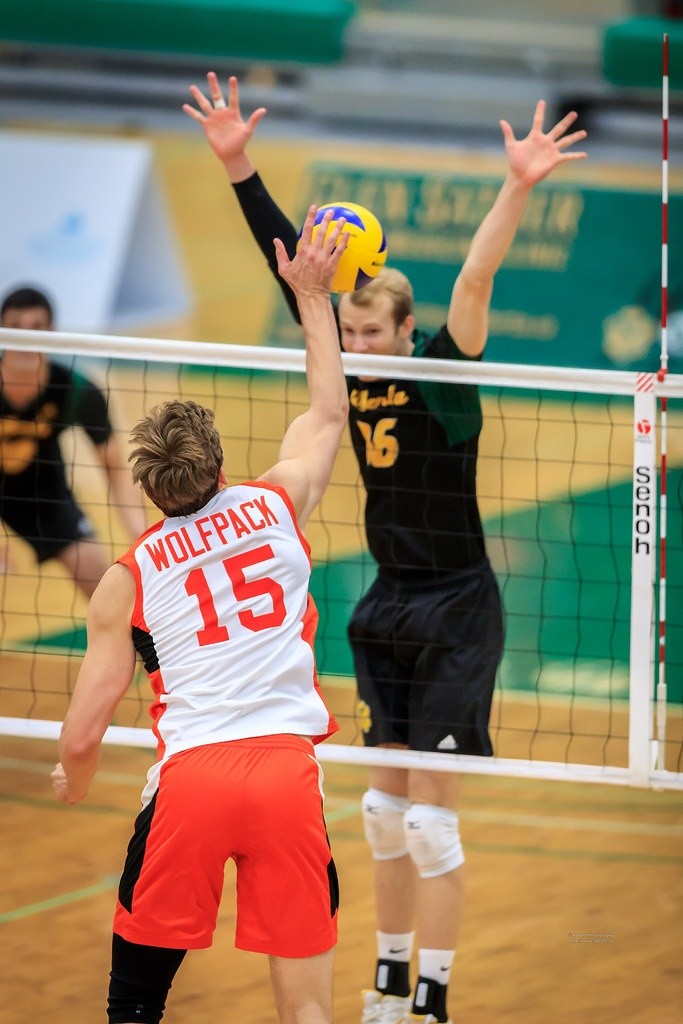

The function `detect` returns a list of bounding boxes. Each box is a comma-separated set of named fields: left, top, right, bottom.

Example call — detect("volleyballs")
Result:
left=296, top=203, right=386, bottom=295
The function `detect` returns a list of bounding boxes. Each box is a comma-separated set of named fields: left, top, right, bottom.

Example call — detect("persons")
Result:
left=166, top=71, right=590, bottom=1023
left=0, top=283, right=156, bottom=619
left=52, top=199, right=363, bottom=1023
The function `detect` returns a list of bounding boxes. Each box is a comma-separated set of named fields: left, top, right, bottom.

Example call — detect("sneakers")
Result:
left=361, top=989, right=452, bottom=1024
